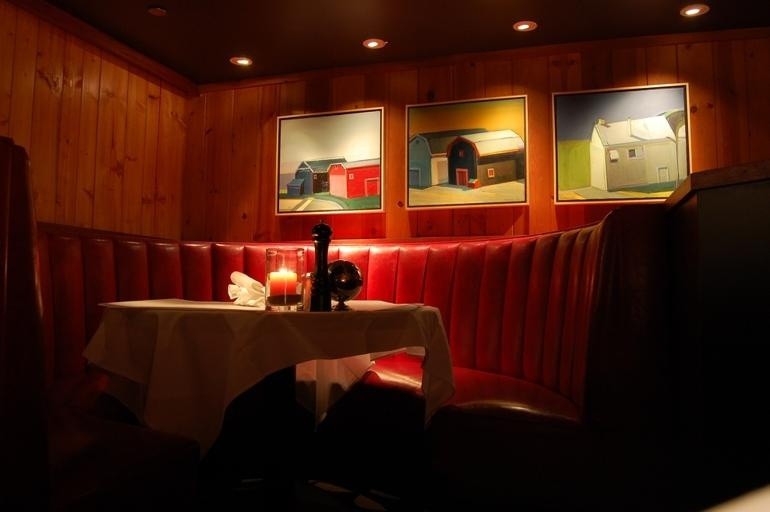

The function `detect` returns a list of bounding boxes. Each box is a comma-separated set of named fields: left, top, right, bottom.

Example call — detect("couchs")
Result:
left=0, top=135, right=671, bottom=492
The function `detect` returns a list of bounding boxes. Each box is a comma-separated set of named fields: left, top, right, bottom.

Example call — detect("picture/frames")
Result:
left=402, top=95, right=531, bottom=209
left=274, top=111, right=387, bottom=216
left=555, top=84, right=692, bottom=205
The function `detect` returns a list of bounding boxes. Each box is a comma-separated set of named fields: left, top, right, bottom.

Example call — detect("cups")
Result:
left=264, top=246, right=306, bottom=312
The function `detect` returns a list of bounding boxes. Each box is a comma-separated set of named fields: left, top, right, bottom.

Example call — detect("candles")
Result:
left=267, top=260, right=299, bottom=304
